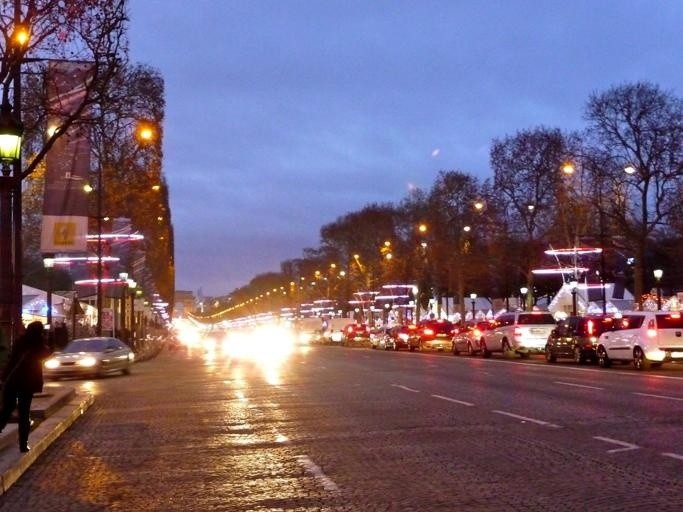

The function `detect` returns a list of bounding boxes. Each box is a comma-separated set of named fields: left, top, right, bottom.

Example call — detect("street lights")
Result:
left=0, top=96, right=25, bottom=352
left=653, top=268, right=664, bottom=311
left=562, top=154, right=636, bottom=315
left=519, top=287, right=528, bottom=310
left=469, top=292, right=479, bottom=320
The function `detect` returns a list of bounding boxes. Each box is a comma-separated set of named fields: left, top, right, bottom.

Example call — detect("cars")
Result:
left=451, top=319, right=496, bottom=357
left=299, top=316, right=454, bottom=353
left=544, top=314, right=614, bottom=365
left=42, top=336, right=136, bottom=382
left=479, top=311, right=558, bottom=360
left=595, top=310, right=683, bottom=371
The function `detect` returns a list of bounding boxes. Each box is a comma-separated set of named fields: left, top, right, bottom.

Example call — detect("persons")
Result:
left=56, top=322, right=68, bottom=349
left=0, top=321, right=56, bottom=453
